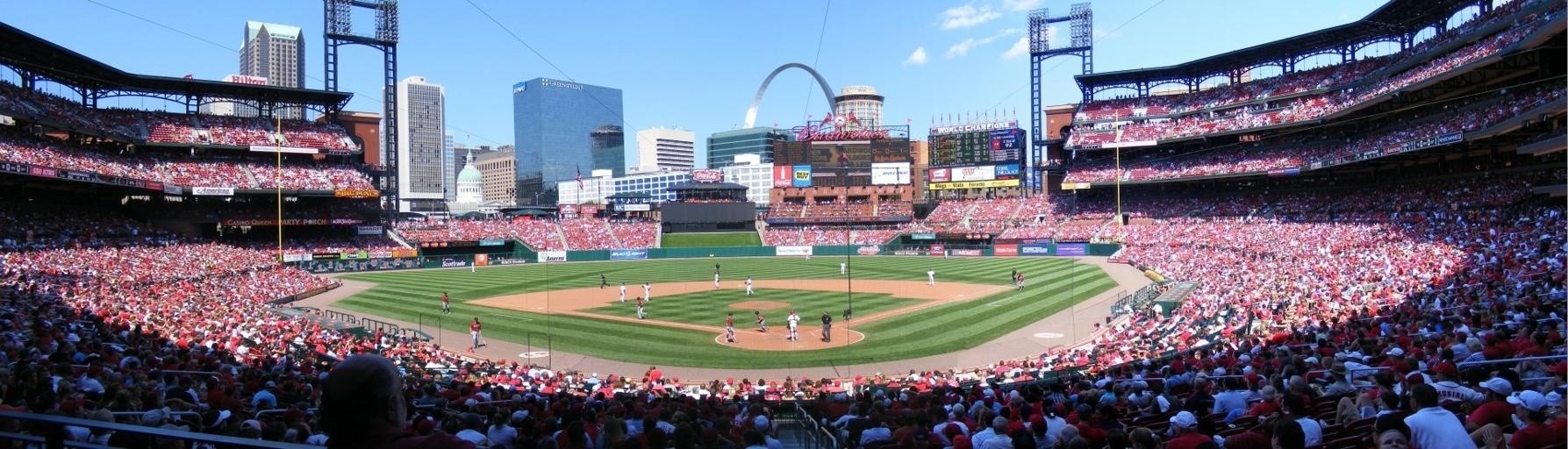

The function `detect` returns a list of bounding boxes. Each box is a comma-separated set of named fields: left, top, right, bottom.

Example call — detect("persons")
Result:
left=0, top=0, right=1566, bottom=449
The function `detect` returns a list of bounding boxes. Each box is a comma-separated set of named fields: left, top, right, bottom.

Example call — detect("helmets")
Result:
left=790, top=310, right=794, bottom=315
left=754, top=311, right=759, bottom=316
